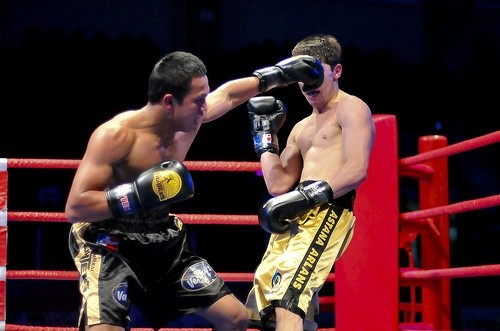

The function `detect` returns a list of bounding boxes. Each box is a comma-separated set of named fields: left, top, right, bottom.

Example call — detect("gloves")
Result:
left=257, top=180, right=333, bottom=233
left=253, top=54, right=325, bottom=92
left=105, top=160, right=194, bottom=221
left=247, top=96, right=286, bottom=159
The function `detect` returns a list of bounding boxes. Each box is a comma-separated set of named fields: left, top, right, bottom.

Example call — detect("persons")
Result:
left=65, top=50, right=324, bottom=331
left=245, top=35, right=376, bottom=331
